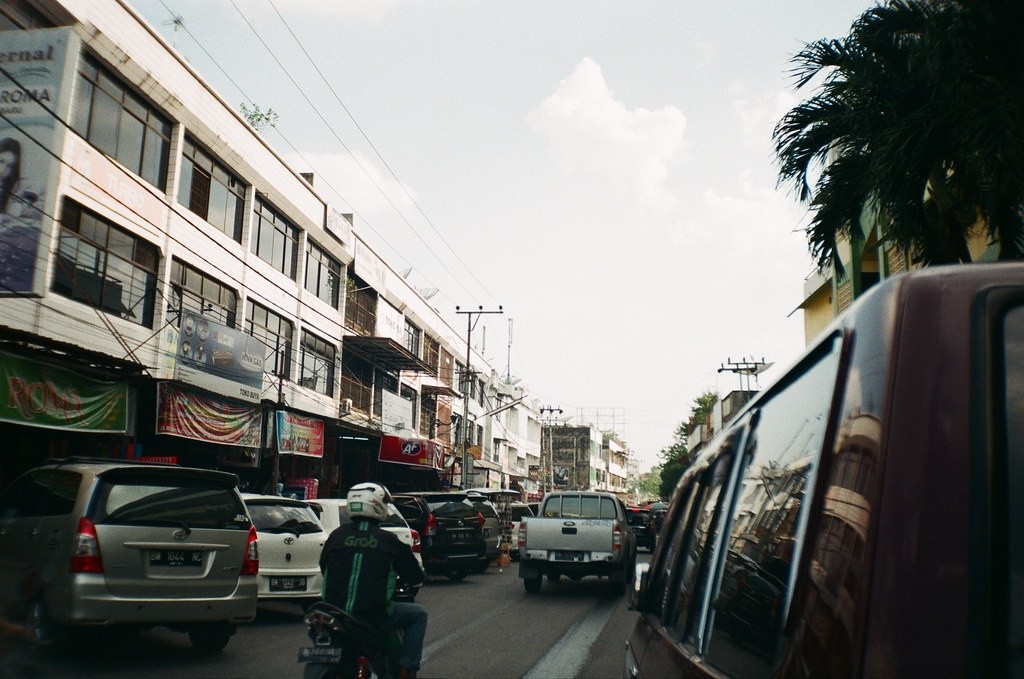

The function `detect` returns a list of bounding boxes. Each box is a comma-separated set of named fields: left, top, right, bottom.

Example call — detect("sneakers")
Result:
left=398, top=667, right=417, bottom=679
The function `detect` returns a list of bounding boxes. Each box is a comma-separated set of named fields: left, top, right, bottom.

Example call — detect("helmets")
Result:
left=346, top=482, right=392, bottom=522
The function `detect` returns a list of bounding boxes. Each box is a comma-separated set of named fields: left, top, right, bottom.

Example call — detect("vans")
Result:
left=622, top=264, right=1022, bottom=677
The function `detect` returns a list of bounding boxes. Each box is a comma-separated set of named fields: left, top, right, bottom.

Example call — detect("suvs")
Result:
left=385, top=491, right=487, bottom=584
left=463, top=489, right=502, bottom=573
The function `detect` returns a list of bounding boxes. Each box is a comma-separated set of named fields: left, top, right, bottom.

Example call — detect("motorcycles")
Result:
left=298, top=571, right=419, bottom=679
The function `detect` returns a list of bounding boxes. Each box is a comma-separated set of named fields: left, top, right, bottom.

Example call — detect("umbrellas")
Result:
left=643, top=500, right=671, bottom=516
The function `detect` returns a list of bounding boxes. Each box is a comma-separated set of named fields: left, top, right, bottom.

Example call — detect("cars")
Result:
left=301, top=498, right=424, bottom=576
left=237, top=491, right=328, bottom=613
left=462, top=484, right=673, bottom=562
left=0, top=459, right=260, bottom=658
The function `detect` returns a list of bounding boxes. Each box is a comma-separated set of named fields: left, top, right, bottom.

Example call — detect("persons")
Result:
left=317, top=480, right=429, bottom=679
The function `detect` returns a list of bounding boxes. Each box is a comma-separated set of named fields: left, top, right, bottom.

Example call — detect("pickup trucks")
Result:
left=517, top=490, right=639, bottom=595
